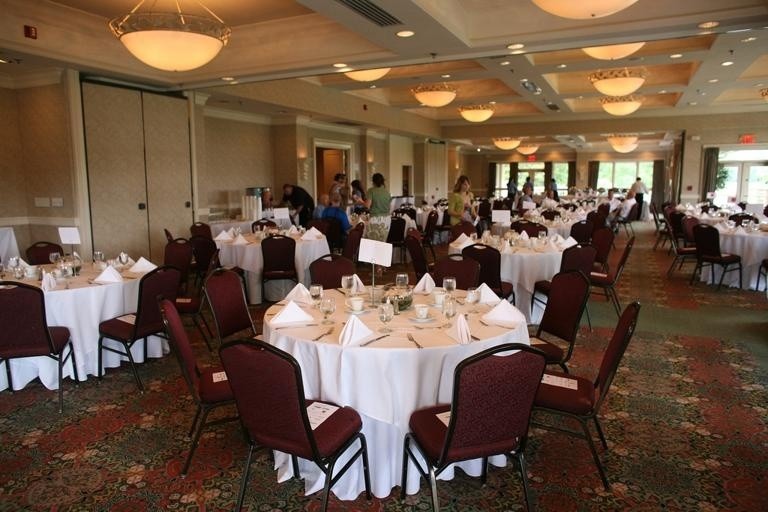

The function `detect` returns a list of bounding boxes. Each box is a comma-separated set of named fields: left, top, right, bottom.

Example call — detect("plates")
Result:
left=409, top=316, right=434, bottom=323
left=345, top=309, right=368, bottom=315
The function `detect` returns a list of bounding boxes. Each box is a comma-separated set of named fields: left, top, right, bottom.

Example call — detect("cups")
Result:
left=415, top=305, right=428, bottom=318
left=433, top=292, right=451, bottom=304
left=344, top=297, right=364, bottom=309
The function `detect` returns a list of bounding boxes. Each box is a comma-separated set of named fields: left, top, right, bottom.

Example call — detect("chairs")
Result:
left=97, top=264, right=182, bottom=391
left=203, top=268, right=257, bottom=347
left=157, top=294, right=237, bottom=476
left=26, top=242, right=64, bottom=265
left=529, top=268, right=591, bottom=375
left=153, top=188, right=768, bottom=305
left=401, top=343, right=547, bottom=510
left=219, top=336, right=373, bottom=510
left=529, top=301, right=641, bottom=489
left=3, top=280, right=79, bottom=414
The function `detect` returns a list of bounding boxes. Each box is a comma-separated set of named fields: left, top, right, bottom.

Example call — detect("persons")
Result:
left=757, top=203, right=768, bottom=293
left=329, top=173, right=348, bottom=212
left=322, top=193, right=353, bottom=245
left=312, top=193, right=329, bottom=219
left=360, top=173, right=392, bottom=277
left=596, top=189, right=622, bottom=213
left=350, top=180, right=366, bottom=213
left=278, top=184, right=314, bottom=227
left=631, top=177, right=648, bottom=221
left=507, top=176, right=558, bottom=215
left=448, top=176, right=478, bottom=229
left=612, top=191, right=637, bottom=233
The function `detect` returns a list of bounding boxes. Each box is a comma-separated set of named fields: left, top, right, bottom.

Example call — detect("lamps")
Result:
left=610, top=133, right=639, bottom=147
left=614, top=146, right=635, bottom=152
left=517, top=144, right=540, bottom=154
left=398, top=30, right=415, bottom=36
left=108, top=1, right=231, bottom=75
left=534, top=0, right=635, bottom=20
left=601, top=97, right=644, bottom=116
left=508, top=44, right=524, bottom=51
left=345, top=67, right=389, bottom=82
left=593, top=71, right=646, bottom=97
left=582, top=42, right=644, bottom=61
left=458, top=104, right=495, bottom=124
left=493, top=138, right=522, bottom=150
left=410, top=83, right=458, bottom=108
left=758, top=87, right=768, bottom=102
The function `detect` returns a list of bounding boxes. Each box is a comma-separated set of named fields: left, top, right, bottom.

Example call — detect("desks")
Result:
left=1, top=262, right=157, bottom=363
left=263, top=283, right=525, bottom=485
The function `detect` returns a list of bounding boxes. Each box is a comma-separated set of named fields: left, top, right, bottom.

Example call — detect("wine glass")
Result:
left=49, top=250, right=60, bottom=268
left=310, top=284, right=324, bottom=310
left=9, top=257, right=18, bottom=274
left=396, top=272, right=408, bottom=294
left=94, top=250, right=102, bottom=268
left=469, top=188, right=601, bottom=253
left=682, top=200, right=762, bottom=233
left=341, top=276, right=355, bottom=300
left=378, top=304, right=394, bottom=333
left=235, top=202, right=448, bottom=242
left=119, top=248, right=128, bottom=266
left=320, top=298, right=336, bottom=324
left=443, top=278, right=456, bottom=298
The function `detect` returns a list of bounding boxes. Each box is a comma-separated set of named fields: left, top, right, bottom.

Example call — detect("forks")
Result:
left=406, top=333, right=423, bottom=348
left=312, top=327, right=335, bottom=341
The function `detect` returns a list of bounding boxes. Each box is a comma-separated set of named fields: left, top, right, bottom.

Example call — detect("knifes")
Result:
left=360, top=334, right=391, bottom=348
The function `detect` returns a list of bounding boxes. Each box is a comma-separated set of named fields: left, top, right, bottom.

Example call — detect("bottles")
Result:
left=386, top=295, right=400, bottom=315
left=36, top=266, right=46, bottom=281
